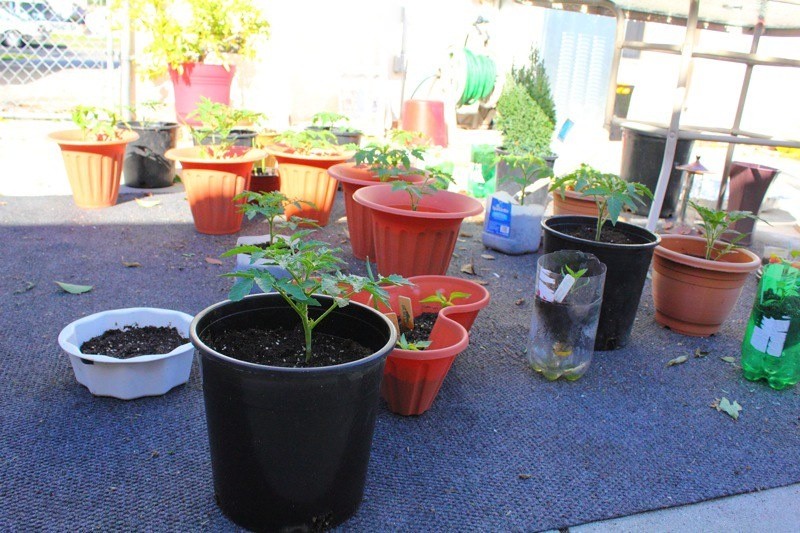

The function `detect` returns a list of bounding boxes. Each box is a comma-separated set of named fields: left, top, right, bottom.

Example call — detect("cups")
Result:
left=718, top=159, right=784, bottom=247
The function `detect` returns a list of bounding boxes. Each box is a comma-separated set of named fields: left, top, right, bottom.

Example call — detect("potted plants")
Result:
left=0, top=0, right=800, bottom=533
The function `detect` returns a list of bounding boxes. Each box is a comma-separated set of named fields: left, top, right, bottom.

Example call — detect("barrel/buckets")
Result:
left=402, top=98, right=448, bottom=151
left=620, top=123, right=698, bottom=220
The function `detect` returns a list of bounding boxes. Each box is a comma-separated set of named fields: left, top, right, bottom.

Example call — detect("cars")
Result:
left=0, top=2, right=90, bottom=48
